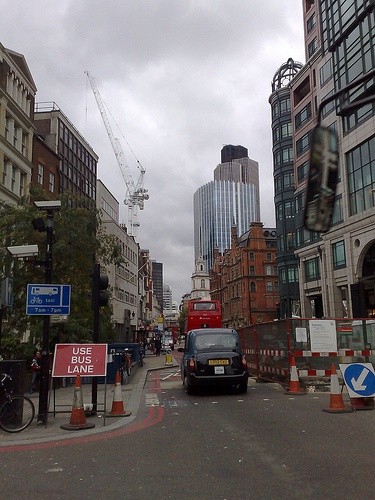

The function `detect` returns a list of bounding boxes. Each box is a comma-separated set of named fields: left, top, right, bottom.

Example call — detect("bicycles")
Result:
left=0, top=371, right=35, bottom=433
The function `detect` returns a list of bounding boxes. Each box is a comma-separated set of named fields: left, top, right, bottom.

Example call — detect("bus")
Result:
left=167, top=326, right=178, bottom=343
left=179, top=300, right=222, bottom=338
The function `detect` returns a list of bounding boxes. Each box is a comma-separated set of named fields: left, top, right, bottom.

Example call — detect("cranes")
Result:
left=82, top=70, right=150, bottom=242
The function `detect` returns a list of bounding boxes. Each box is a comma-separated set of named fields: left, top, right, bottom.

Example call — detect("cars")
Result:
left=177, top=329, right=249, bottom=396
left=177, top=335, right=185, bottom=352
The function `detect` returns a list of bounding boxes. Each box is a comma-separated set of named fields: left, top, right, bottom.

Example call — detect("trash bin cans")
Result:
left=0, top=360, right=30, bottom=426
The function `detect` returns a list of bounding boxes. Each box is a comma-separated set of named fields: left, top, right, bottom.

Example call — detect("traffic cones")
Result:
left=60, top=373, right=95, bottom=430
left=347, top=398, right=364, bottom=407
left=103, top=372, right=131, bottom=418
left=323, top=362, right=353, bottom=413
left=284, top=356, right=307, bottom=394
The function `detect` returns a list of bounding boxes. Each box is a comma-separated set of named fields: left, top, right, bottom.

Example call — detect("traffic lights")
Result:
left=99, top=276, right=108, bottom=308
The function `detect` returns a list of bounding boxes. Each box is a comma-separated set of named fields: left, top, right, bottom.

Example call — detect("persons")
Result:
left=150, top=337, right=155, bottom=352
left=155, top=337, right=162, bottom=357
left=28, top=350, right=41, bottom=394
